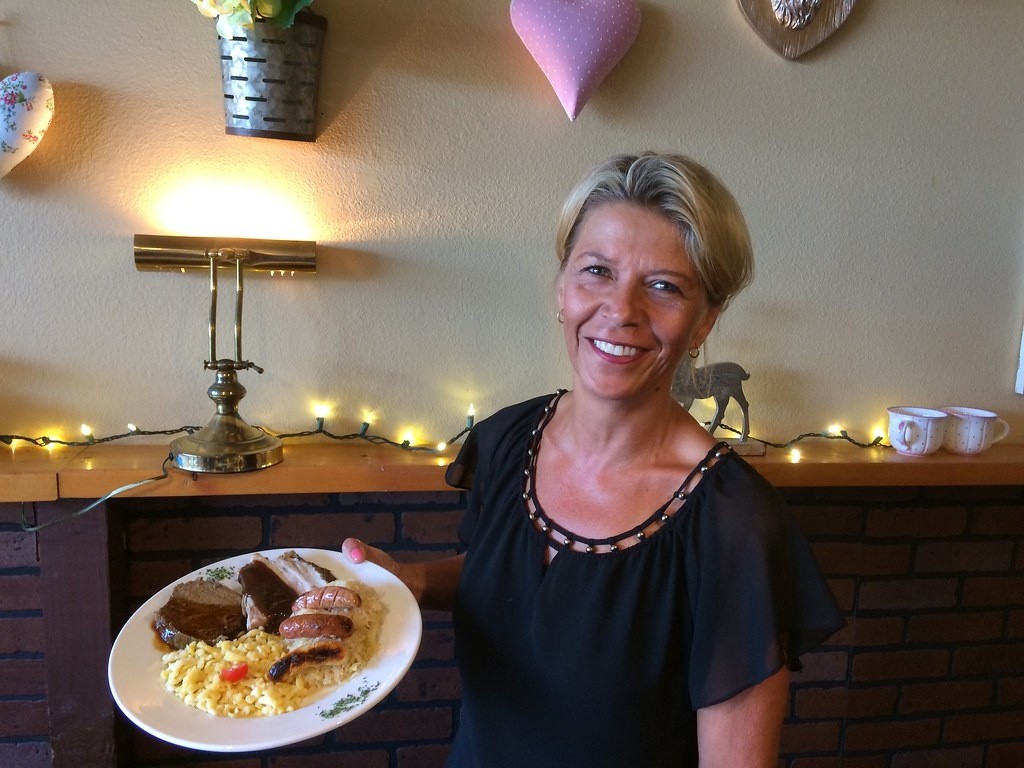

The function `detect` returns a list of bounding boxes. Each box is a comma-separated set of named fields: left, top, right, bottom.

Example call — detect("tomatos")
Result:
left=222, top=662, right=248, bottom=681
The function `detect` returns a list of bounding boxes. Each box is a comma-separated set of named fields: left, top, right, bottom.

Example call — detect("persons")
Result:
left=340, top=149, right=846, bottom=768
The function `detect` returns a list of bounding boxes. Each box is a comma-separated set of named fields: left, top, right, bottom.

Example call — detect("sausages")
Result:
left=268, top=585, right=362, bottom=681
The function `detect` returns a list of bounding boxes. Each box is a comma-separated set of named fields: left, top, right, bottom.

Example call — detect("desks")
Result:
left=0, top=446, right=1024, bottom=768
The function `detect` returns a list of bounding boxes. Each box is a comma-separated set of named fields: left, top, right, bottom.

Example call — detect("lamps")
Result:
left=134, top=233, right=315, bottom=473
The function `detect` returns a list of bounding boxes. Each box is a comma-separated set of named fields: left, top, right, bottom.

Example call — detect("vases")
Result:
left=212, top=10, right=326, bottom=142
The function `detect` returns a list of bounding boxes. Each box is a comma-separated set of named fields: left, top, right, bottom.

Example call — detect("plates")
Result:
left=107, top=549, right=424, bottom=752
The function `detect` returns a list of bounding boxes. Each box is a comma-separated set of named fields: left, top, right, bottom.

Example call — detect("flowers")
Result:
left=192, top=0, right=315, bottom=40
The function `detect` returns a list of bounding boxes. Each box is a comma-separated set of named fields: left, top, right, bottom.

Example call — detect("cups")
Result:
left=886, top=405, right=948, bottom=457
left=940, top=407, right=1010, bottom=457
